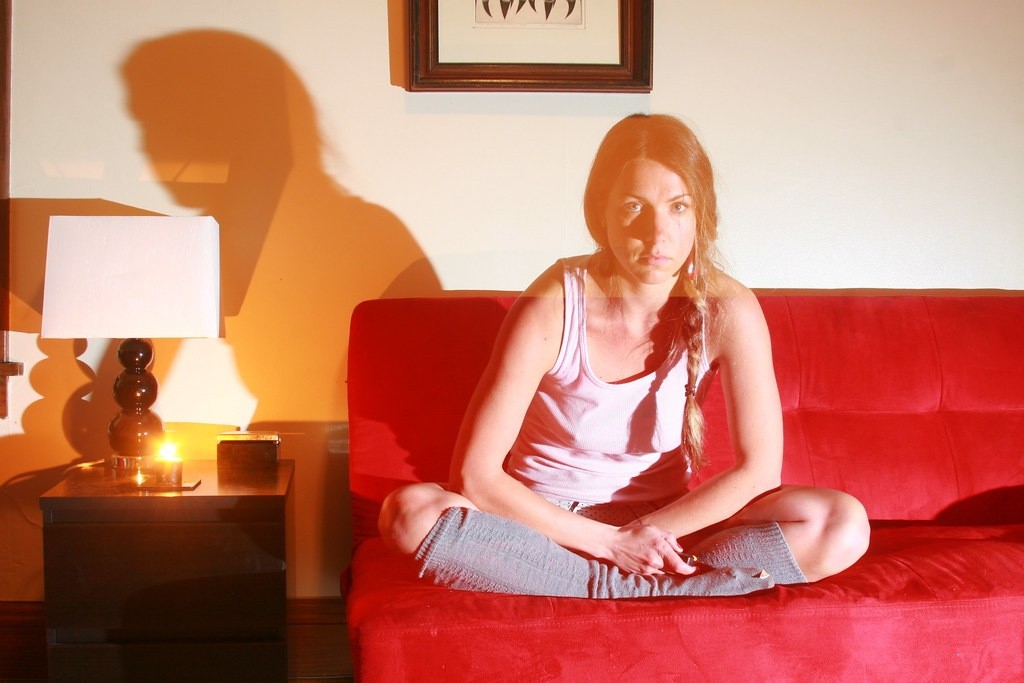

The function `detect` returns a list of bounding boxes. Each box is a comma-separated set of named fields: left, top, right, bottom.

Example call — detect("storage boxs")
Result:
left=217, top=432, right=280, bottom=476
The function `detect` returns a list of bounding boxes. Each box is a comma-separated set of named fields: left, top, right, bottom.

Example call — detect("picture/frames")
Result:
left=407, top=0, right=654, bottom=94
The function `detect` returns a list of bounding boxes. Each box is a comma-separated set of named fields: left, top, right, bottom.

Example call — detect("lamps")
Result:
left=40, top=215, right=220, bottom=474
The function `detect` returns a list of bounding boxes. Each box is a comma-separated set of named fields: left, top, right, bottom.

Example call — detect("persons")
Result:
left=377, top=113, right=871, bottom=600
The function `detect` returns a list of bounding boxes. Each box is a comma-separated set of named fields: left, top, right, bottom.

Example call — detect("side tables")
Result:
left=38, top=459, right=295, bottom=683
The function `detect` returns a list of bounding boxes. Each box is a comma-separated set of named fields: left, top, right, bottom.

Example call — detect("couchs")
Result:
left=345, top=295, right=1023, bottom=683
